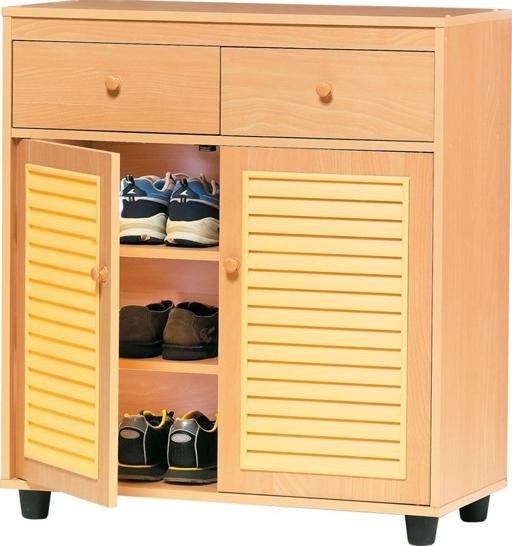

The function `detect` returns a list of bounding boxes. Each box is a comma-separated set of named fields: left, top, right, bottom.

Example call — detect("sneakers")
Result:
left=119, top=171, right=177, bottom=246
left=117, top=412, right=174, bottom=481
left=164, top=409, right=218, bottom=486
left=163, top=175, right=221, bottom=245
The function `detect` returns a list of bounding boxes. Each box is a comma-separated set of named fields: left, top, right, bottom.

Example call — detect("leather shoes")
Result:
left=162, top=300, right=218, bottom=361
left=119, top=301, right=173, bottom=359
